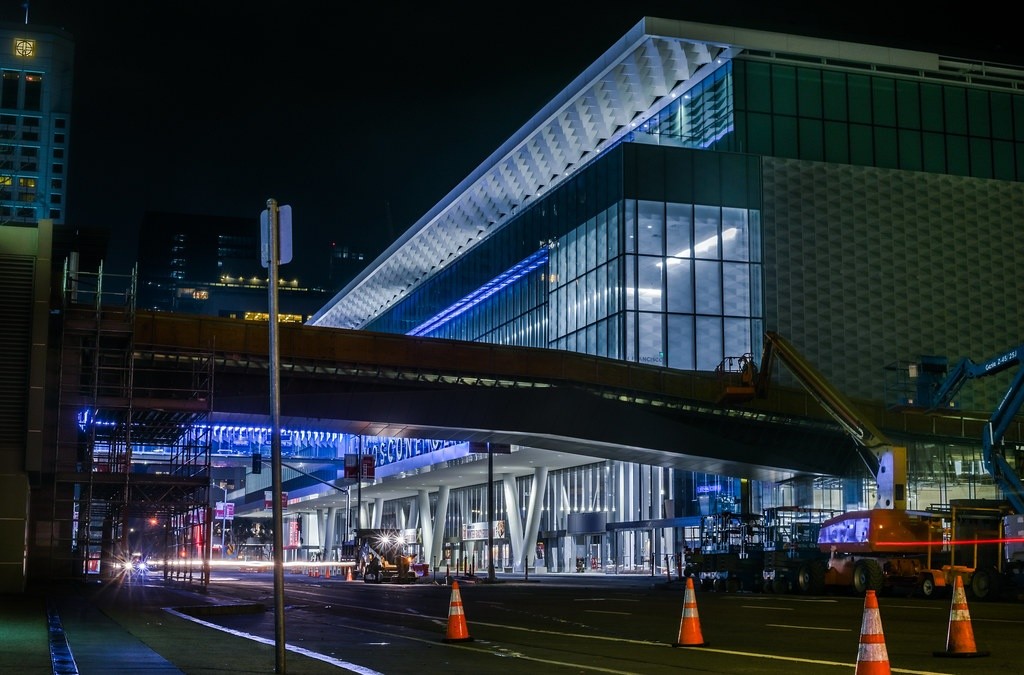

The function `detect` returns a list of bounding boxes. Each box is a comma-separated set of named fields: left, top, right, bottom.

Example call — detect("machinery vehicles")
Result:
left=716, top=329, right=945, bottom=596
left=341, top=528, right=417, bottom=583
left=883, top=346, right=1024, bottom=599
left=685, top=504, right=846, bottom=596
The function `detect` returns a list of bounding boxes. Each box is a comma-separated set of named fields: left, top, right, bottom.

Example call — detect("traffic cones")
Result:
left=933, top=575, right=991, bottom=658
left=854, top=588, right=893, bottom=675
left=345, top=566, right=353, bottom=582
left=308, top=567, right=313, bottom=576
left=671, top=577, right=711, bottom=649
left=314, top=567, right=320, bottom=577
left=325, top=566, right=331, bottom=579
left=440, top=582, right=474, bottom=643
